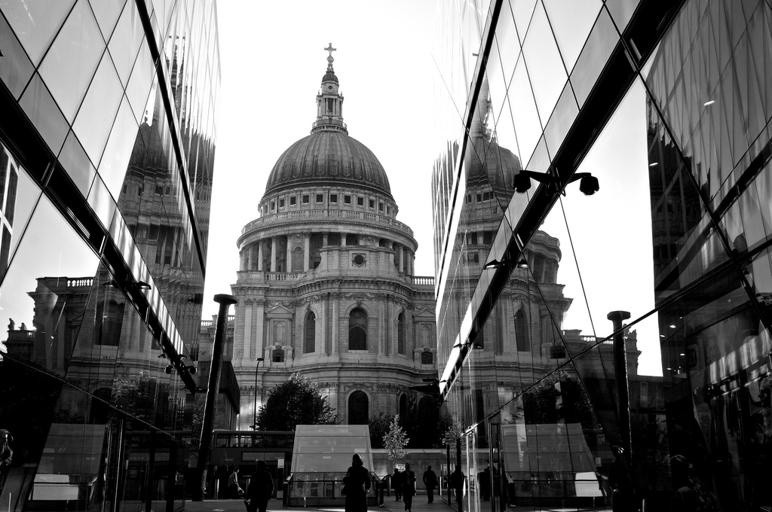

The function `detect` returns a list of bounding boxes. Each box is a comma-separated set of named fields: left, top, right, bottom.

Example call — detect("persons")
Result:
left=226, top=467, right=245, bottom=499
left=423, top=465, right=437, bottom=504
left=391, top=462, right=416, bottom=512
left=200, top=464, right=229, bottom=499
left=341, top=453, right=371, bottom=512
left=243, top=460, right=272, bottom=512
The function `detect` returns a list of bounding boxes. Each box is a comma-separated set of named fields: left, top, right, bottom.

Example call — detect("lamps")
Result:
left=131, top=280, right=152, bottom=291
left=566, top=171, right=601, bottom=195
left=516, top=258, right=528, bottom=269
left=103, top=281, right=117, bottom=288
left=513, top=168, right=555, bottom=196
left=484, top=258, right=500, bottom=270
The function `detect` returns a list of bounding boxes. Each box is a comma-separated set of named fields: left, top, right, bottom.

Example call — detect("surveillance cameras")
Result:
left=166, top=367, right=171, bottom=373
left=511, top=173, right=531, bottom=193
left=579, top=176, right=599, bottom=195
left=190, top=366, right=198, bottom=374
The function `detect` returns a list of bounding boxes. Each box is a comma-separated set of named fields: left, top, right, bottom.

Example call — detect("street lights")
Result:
left=252, top=357, right=265, bottom=430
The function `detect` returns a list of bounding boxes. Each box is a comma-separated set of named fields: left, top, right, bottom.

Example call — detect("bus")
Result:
left=124, top=430, right=193, bottom=478
left=207, top=428, right=295, bottom=466
